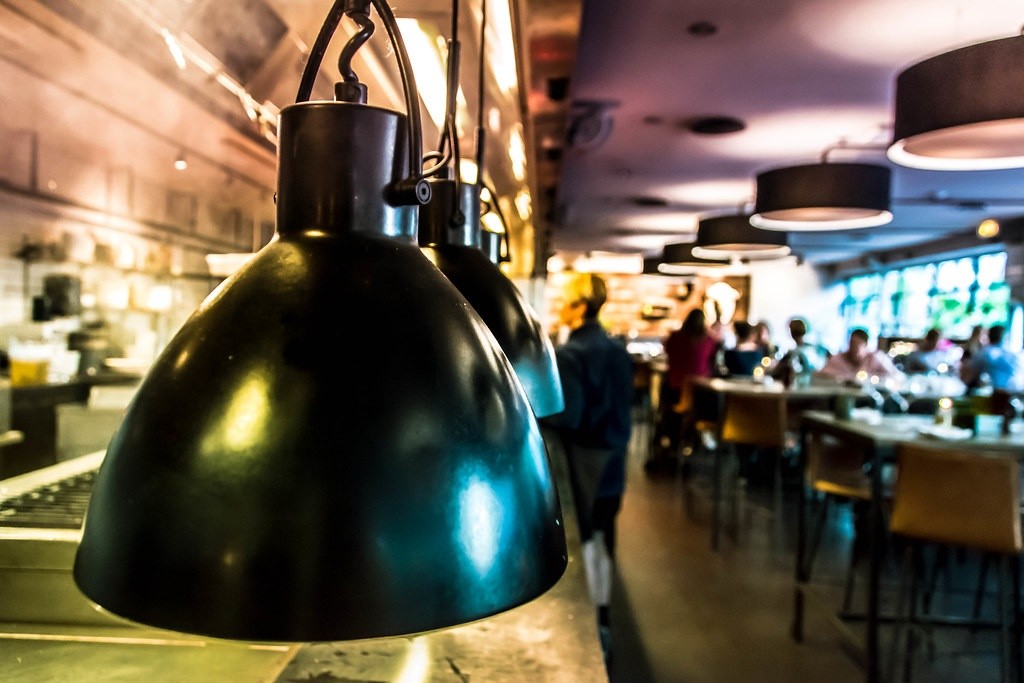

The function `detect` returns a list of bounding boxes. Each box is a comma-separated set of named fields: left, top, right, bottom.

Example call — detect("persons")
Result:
left=647, top=293, right=1024, bottom=488
left=540, top=270, right=634, bottom=634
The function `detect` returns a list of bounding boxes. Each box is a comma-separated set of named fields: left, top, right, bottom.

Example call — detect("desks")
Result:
left=791, top=410, right=1024, bottom=683
left=678, top=374, right=997, bottom=553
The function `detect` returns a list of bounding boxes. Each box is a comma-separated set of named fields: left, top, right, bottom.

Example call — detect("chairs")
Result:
left=880, top=442, right=1024, bottom=683
left=721, top=380, right=798, bottom=546
left=807, top=432, right=932, bottom=614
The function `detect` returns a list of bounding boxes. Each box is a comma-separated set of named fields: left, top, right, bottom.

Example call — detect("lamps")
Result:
left=477, top=0, right=512, bottom=265
left=691, top=212, right=793, bottom=261
left=748, top=145, right=898, bottom=233
left=657, top=243, right=732, bottom=276
left=640, top=257, right=698, bottom=279
left=419, top=0, right=566, bottom=419
left=70, top=0, right=566, bottom=645
left=884, top=32, right=1024, bottom=172
